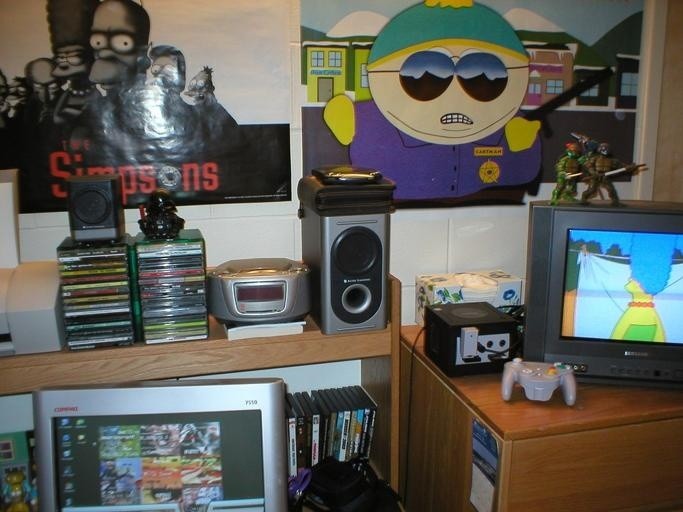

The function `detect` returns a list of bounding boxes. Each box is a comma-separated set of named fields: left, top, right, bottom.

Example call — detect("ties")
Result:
left=33, top=378, right=288, bottom=512
left=523, top=200, right=683, bottom=390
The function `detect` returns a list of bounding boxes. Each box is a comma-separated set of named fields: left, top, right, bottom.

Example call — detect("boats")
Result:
left=66, top=175, right=124, bottom=246
left=300, top=202, right=390, bottom=336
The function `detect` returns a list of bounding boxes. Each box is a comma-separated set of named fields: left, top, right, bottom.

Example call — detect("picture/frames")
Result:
left=399, top=323, right=683, bottom=511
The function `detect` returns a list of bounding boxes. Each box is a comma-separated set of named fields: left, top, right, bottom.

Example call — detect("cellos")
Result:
left=0, top=168, right=67, bottom=357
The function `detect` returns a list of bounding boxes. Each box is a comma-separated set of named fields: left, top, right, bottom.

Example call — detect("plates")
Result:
left=1, top=271, right=402, bottom=511
